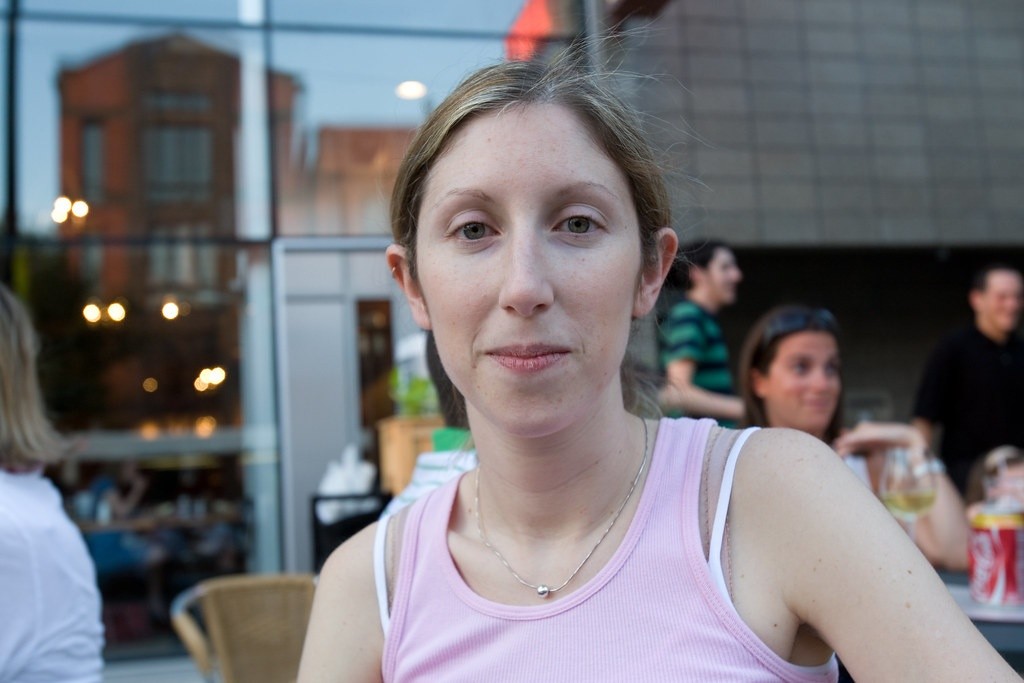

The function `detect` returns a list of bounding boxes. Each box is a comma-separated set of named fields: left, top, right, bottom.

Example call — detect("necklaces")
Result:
left=476, top=415, right=648, bottom=598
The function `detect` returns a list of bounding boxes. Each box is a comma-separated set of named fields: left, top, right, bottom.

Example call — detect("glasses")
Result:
left=758, top=308, right=841, bottom=366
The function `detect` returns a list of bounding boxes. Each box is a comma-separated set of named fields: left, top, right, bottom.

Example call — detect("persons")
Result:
left=657, top=239, right=746, bottom=430
left=912, top=268, right=1024, bottom=498
left=0, top=287, right=105, bottom=683
left=294, top=61, right=1024, bottom=683
left=86, top=455, right=258, bottom=590
left=740, top=303, right=973, bottom=571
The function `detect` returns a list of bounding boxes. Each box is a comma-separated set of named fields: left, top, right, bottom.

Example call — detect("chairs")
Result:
left=170, top=575, right=318, bottom=683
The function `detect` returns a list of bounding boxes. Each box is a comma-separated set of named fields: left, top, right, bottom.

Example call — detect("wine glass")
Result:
left=879, top=446, right=936, bottom=545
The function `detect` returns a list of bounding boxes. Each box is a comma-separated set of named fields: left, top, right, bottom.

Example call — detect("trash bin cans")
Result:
left=311, top=491, right=392, bottom=574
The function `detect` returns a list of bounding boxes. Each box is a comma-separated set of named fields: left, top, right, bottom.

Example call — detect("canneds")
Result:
left=967, top=506, right=1024, bottom=607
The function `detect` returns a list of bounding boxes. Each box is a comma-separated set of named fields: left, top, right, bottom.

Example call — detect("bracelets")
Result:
left=913, top=458, right=946, bottom=478
